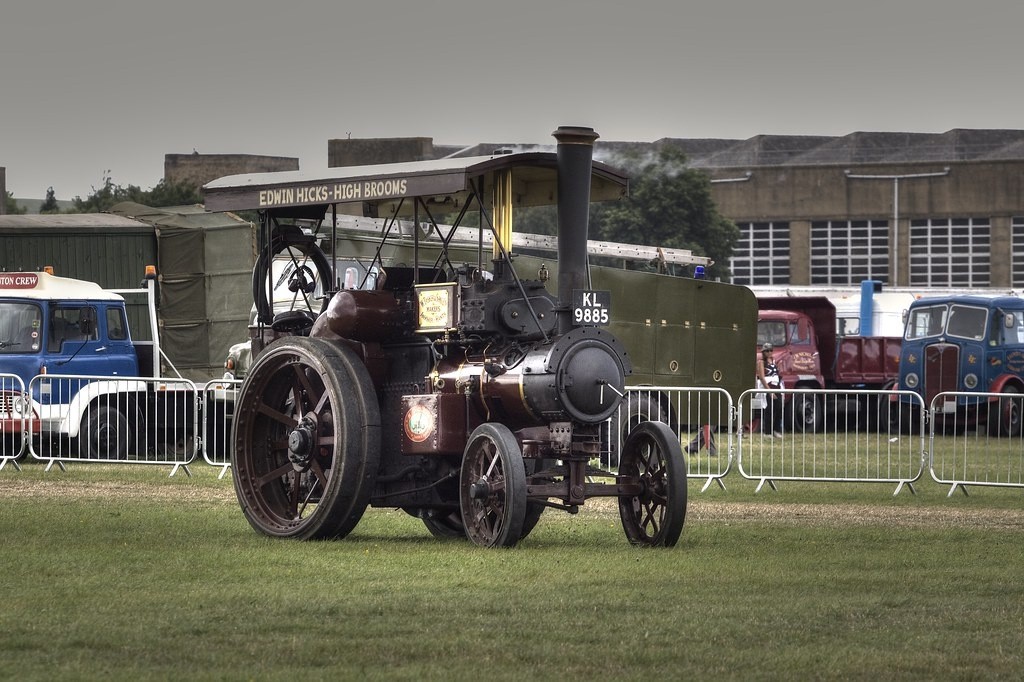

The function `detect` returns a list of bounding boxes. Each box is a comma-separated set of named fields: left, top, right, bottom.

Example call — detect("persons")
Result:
left=684, top=424, right=718, bottom=457
left=759, top=343, right=785, bottom=438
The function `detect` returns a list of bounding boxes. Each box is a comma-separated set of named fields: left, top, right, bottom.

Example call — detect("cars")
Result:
left=876, top=295, right=1024, bottom=438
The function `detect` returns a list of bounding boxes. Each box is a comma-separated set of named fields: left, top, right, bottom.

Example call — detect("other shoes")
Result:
left=764, top=431, right=782, bottom=439
left=684, top=446, right=696, bottom=458
left=706, top=453, right=718, bottom=458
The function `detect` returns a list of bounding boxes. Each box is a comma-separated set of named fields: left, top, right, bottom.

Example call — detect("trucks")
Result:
left=1, top=264, right=229, bottom=460
left=754, top=294, right=912, bottom=435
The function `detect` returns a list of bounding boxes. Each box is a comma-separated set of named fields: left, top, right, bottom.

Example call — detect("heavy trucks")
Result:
left=216, top=232, right=756, bottom=472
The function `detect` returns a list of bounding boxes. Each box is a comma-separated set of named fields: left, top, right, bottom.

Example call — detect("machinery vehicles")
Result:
left=200, top=123, right=687, bottom=554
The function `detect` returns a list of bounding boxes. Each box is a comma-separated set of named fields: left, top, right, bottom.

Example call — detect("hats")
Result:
left=761, top=343, right=773, bottom=351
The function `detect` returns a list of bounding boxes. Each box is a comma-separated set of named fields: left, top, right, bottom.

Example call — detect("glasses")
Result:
left=763, top=350, right=772, bottom=352
